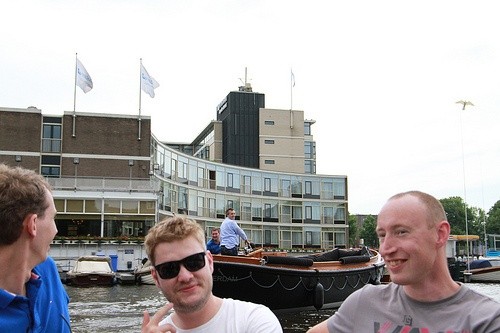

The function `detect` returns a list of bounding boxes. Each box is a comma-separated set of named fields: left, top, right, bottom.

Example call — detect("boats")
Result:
left=62, top=250, right=115, bottom=287
left=133, top=257, right=159, bottom=285
left=212, top=243, right=389, bottom=319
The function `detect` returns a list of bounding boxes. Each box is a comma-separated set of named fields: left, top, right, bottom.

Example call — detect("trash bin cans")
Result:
left=96, top=255, right=118, bottom=271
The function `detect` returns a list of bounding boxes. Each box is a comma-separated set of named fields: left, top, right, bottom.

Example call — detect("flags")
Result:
left=76, top=57, right=93, bottom=94
left=292, top=71, right=296, bottom=87
left=141, top=63, right=160, bottom=98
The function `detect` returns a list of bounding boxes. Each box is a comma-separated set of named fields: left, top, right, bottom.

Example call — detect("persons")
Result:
left=141, top=214, right=284, bottom=333
left=220, top=207, right=250, bottom=256
left=306, top=191, right=500, bottom=333
left=207, top=228, right=220, bottom=254
left=0, top=159, right=72, bottom=333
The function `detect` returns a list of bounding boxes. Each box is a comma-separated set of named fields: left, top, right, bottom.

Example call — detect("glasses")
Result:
left=154, top=252, right=206, bottom=279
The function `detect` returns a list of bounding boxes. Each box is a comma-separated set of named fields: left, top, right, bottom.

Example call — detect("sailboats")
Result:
left=447, top=162, right=500, bottom=284
